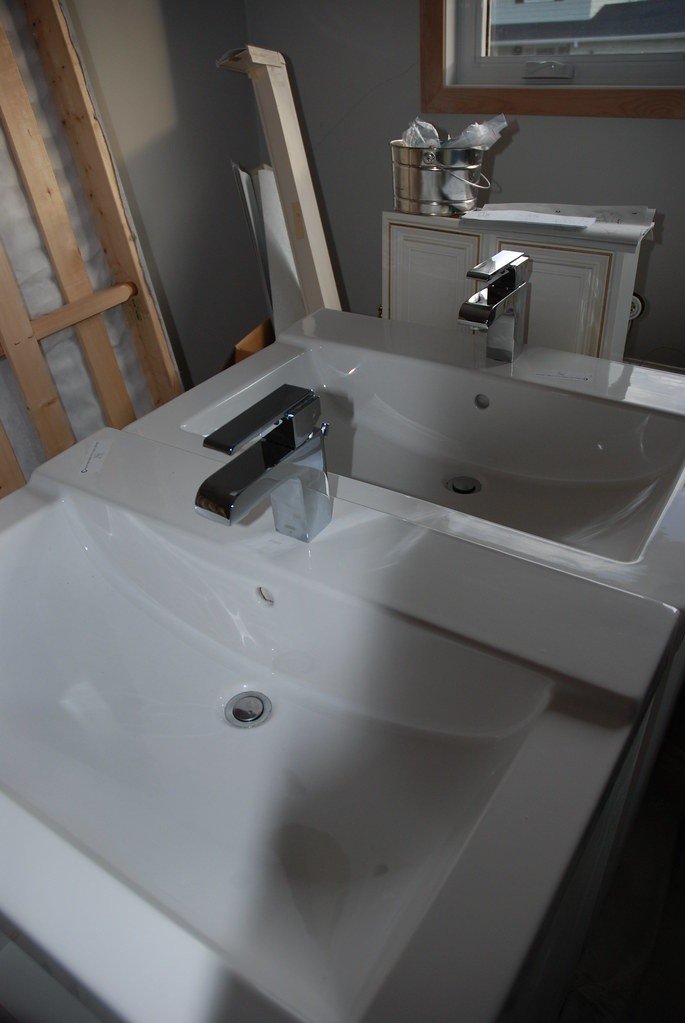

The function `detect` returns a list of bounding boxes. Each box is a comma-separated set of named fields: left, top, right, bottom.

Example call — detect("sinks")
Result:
left=118, top=335, right=685, bottom=701
left=1, top=483, right=568, bottom=1022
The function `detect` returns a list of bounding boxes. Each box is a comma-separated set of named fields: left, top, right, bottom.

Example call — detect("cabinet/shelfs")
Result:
left=380, top=208, right=644, bottom=365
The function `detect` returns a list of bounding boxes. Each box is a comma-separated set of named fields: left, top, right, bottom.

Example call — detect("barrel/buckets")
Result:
left=390, top=139, right=490, bottom=216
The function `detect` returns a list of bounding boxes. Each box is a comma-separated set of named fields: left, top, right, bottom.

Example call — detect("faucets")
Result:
left=193, top=383, right=333, bottom=544
left=457, top=249, right=529, bottom=363
